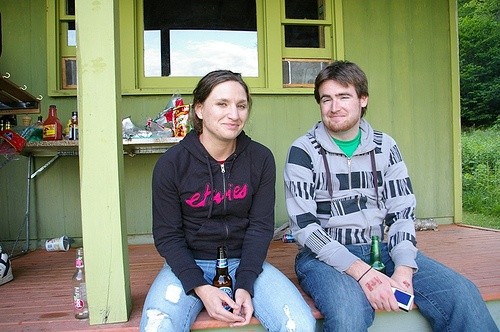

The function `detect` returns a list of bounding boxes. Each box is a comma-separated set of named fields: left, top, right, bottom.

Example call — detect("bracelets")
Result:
left=356, top=266, right=373, bottom=282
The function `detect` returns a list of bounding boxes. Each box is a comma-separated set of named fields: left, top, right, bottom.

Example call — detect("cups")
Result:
left=46, top=235, right=70, bottom=252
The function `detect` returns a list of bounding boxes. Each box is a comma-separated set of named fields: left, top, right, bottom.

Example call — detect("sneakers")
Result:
left=0, top=252, right=14, bottom=286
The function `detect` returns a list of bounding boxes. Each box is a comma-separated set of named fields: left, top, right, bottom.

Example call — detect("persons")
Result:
left=138, top=69, right=316, bottom=332
left=284, top=61, right=500, bottom=332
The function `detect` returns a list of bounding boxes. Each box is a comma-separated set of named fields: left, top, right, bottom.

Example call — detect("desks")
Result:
left=9, top=137, right=184, bottom=259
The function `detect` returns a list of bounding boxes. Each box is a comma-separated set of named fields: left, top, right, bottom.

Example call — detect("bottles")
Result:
left=282, top=233, right=296, bottom=242
left=69, top=112, right=78, bottom=140
left=213, top=247, right=234, bottom=314
left=72, top=248, right=89, bottom=319
left=369, top=235, right=386, bottom=275
left=43, top=105, right=63, bottom=141
